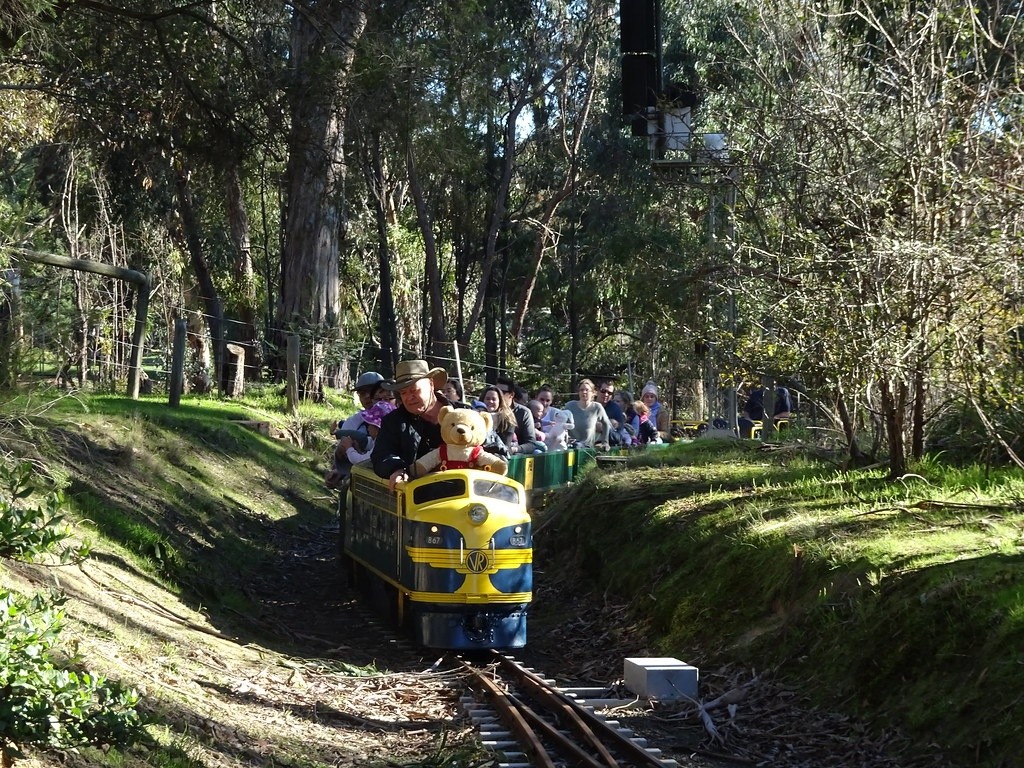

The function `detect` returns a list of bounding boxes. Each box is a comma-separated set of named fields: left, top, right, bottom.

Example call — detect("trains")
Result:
left=339, top=428, right=703, bottom=654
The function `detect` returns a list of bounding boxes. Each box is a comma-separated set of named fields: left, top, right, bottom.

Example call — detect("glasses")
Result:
left=357, top=388, right=378, bottom=396
left=502, top=390, right=512, bottom=394
left=599, top=389, right=613, bottom=395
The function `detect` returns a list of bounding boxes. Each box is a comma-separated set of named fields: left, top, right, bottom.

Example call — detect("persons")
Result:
left=744, top=385, right=794, bottom=437
left=340, top=401, right=397, bottom=470
left=370, top=361, right=507, bottom=492
left=330, top=421, right=352, bottom=522
left=325, top=371, right=397, bottom=490
left=440, top=378, right=669, bottom=460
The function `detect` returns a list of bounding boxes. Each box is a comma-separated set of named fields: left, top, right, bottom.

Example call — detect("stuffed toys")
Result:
left=410, top=405, right=508, bottom=477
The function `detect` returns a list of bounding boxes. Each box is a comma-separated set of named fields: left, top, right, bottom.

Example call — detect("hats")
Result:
left=381, top=360, right=448, bottom=391
left=351, top=372, right=385, bottom=391
left=640, top=381, right=658, bottom=400
left=471, top=399, right=487, bottom=412
left=360, top=401, right=398, bottom=428
